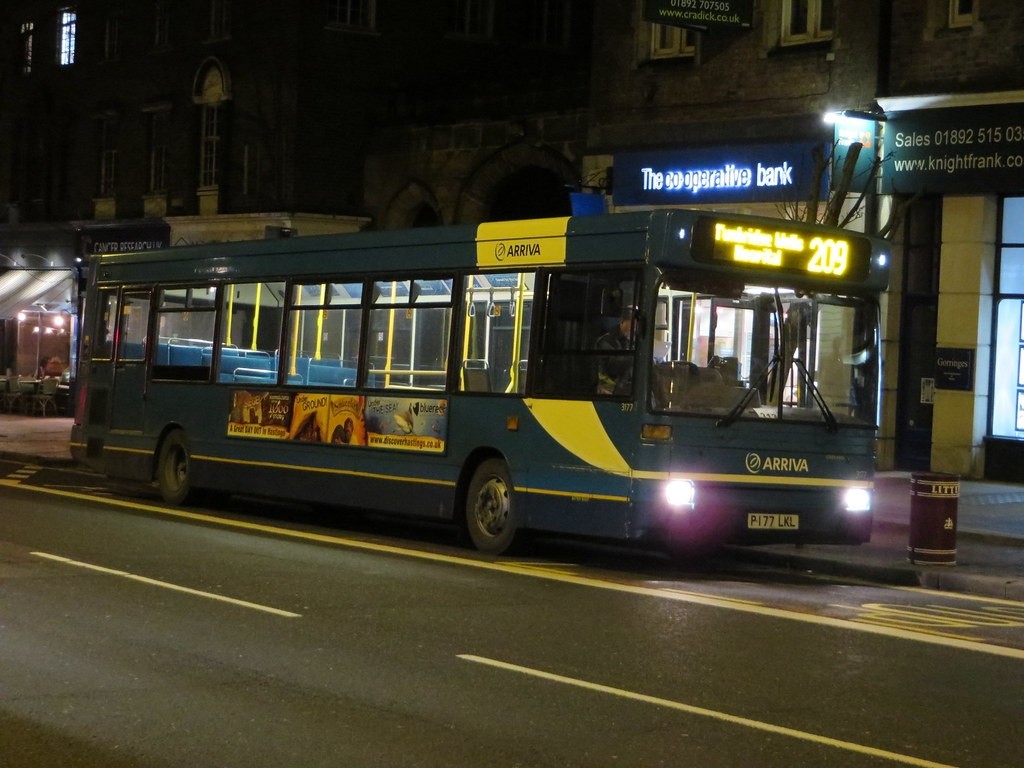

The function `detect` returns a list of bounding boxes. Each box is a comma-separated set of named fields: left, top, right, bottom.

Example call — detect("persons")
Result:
left=596, top=304, right=638, bottom=395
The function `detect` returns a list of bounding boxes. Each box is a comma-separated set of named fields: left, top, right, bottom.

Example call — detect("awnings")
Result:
left=0, top=265, right=79, bottom=322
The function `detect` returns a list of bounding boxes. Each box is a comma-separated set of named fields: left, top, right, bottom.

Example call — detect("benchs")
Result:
left=104, top=336, right=377, bottom=387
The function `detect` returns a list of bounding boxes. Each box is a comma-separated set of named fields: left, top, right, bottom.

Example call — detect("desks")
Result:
left=0, top=379, right=41, bottom=412
left=56, top=386, right=70, bottom=408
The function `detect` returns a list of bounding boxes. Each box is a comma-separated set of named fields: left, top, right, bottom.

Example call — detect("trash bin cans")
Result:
left=906, top=471, right=958, bottom=567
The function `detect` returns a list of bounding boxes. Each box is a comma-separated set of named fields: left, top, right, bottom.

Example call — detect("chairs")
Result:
left=464, top=360, right=493, bottom=395
left=5, top=377, right=29, bottom=413
left=27, top=380, right=57, bottom=416
left=518, top=359, right=529, bottom=394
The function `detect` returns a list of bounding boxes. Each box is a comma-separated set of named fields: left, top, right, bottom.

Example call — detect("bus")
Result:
left=67, top=209, right=891, bottom=571
